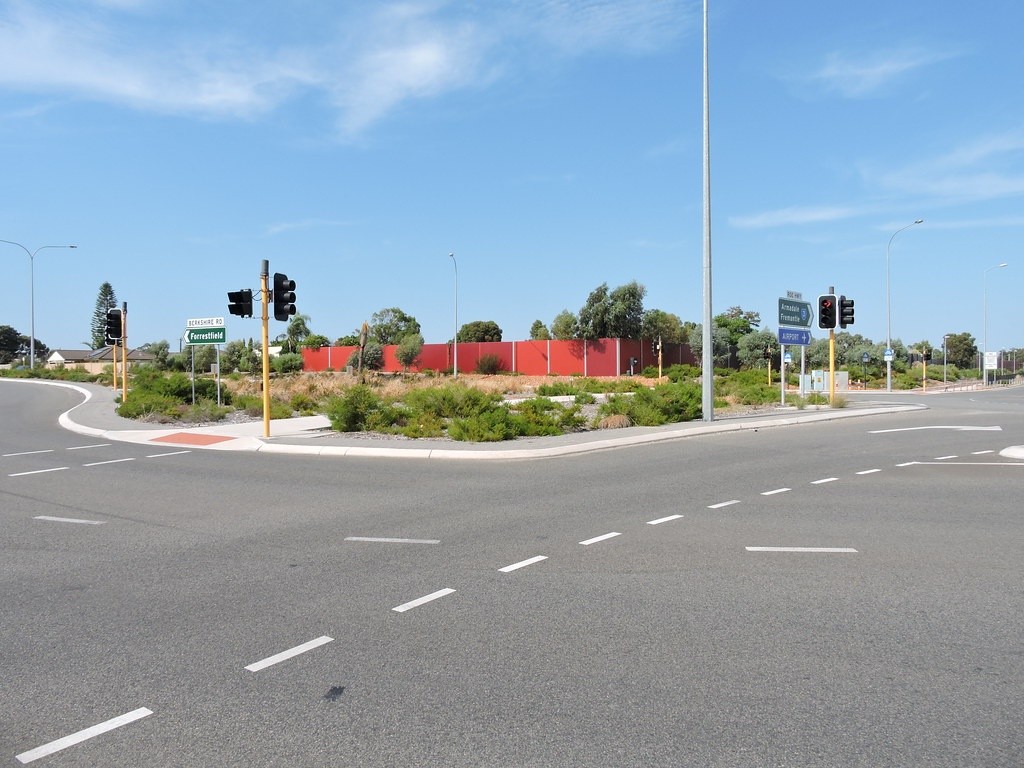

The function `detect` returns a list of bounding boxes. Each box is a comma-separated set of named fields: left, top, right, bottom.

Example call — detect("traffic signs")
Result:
left=777, top=297, right=814, bottom=327
left=777, top=325, right=813, bottom=345
left=182, top=328, right=226, bottom=345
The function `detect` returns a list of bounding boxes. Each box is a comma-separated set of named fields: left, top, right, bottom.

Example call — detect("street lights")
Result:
left=944, top=336, right=950, bottom=385
left=1014, top=350, right=1018, bottom=373
left=984, top=263, right=1008, bottom=387
left=0, top=239, right=77, bottom=371
left=884, top=219, right=923, bottom=390
left=449, top=252, right=458, bottom=379
left=978, top=343, right=983, bottom=377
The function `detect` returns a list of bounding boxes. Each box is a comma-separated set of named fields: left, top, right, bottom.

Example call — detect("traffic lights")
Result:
left=762, top=348, right=767, bottom=359
left=274, top=273, right=296, bottom=322
left=652, top=341, right=659, bottom=357
left=105, top=326, right=115, bottom=347
left=924, top=348, right=932, bottom=359
left=817, top=294, right=839, bottom=330
left=227, top=289, right=253, bottom=319
left=838, top=296, right=855, bottom=329
left=106, top=309, right=122, bottom=340
